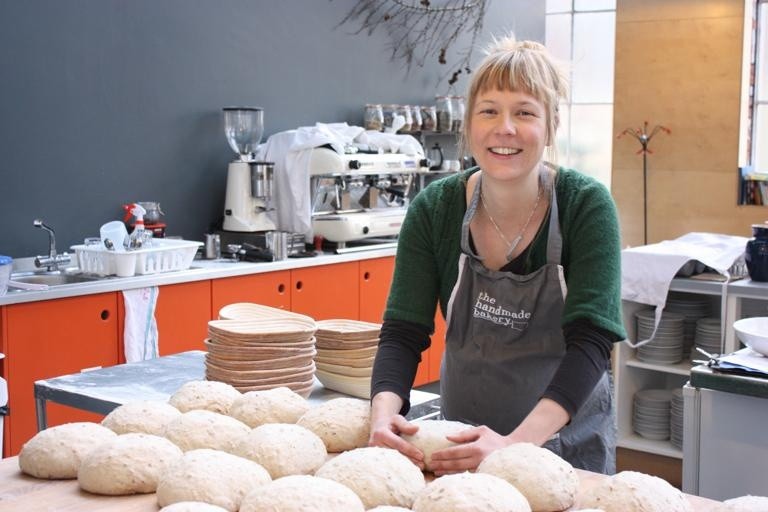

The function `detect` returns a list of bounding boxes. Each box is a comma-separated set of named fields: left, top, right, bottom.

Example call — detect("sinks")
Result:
left=6, top=271, right=99, bottom=289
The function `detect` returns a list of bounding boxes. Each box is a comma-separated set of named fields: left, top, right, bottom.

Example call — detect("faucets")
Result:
left=33, top=215, right=58, bottom=269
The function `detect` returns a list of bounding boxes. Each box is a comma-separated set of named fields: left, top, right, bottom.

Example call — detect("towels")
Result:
left=119, top=285, right=161, bottom=364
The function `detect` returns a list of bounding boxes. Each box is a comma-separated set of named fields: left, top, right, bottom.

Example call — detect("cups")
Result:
left=204, top=232, right=221, bottom=259
left=266, top=231, right=293, bottom=262
left=100, top=220, right=130, bottom=251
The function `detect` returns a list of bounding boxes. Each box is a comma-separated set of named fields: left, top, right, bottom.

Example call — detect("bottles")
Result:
left=363, top=104, right=437, bottom=131
left=436, top=95, right=465, bottom=133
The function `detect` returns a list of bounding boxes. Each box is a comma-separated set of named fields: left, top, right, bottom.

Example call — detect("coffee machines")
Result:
left=219, top=105, right=316, bottom=262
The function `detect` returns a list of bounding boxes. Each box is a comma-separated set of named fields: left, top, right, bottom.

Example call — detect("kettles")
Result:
left=429, top=142, right=446, bottom=172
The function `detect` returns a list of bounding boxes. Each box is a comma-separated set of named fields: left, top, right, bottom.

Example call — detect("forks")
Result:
left=123, top=235, right=130, bottom=251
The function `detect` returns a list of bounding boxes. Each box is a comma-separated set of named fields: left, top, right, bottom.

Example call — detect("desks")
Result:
left=32, top=347, right=445, bottom=429
left=1, top=418, right=725, bottom=510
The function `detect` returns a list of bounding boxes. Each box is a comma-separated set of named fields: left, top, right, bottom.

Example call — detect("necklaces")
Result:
left=477, top=172, right=545, bottom=264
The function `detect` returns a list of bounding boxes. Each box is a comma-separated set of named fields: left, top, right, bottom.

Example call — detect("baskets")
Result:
left=69, top=237, right=205, bottom=278
left=204, top=316, right=319, bottom=401
left=315, top=319, right=383, bottom=400
left=219, top=302, right=319, bottom=333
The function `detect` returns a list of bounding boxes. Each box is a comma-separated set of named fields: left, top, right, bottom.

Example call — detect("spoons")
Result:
left=104, top=239, right=115, bottom=251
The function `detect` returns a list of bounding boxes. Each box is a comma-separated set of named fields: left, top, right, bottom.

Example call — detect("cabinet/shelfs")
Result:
left=610, top=274, right=766, bottom=461
left=1, top=278, right=212, bottom=460
left=371, top=129, right=466, bottom=193
left=360, top=243, right=431, bottom=390
left=429, top=300, right=449, bottom=383
left=677, top=356, right=765, bottom=502
left=213, top=262, right=361, bottom=332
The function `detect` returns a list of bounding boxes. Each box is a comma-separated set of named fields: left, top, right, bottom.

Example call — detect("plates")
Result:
left=632, top=385, right=684, bottom=451
left=634, top=297, right=722, bottom=370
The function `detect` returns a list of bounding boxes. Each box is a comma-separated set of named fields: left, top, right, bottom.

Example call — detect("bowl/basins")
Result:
left=732, top=315, right=768, bottom=359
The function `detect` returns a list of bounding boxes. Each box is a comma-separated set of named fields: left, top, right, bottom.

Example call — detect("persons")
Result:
left=368, top=41, right=627, bottom=479
